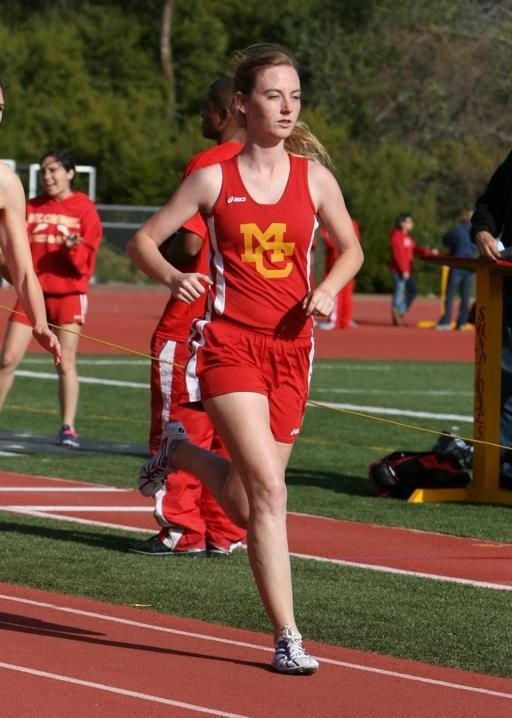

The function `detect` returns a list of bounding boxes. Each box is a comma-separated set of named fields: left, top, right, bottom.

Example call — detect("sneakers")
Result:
left=272, top=630, right=320, bottom=674
left=127, top=533, right=230, bottom=557
left=138, top=420, right=188, bottom=497
left=60, top=423, right=79, bottom=447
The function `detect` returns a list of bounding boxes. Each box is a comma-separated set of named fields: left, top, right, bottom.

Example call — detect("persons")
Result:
left=124, top=40, right=365, bottom=675
left=124, top=76, right=252, bottom=555
left=467, top=150, right=512, bottom=493
left=1, top=148, right=102, bottom=446
left=389, top=212, right=440, bottom=326
left=432, top=201, right=479, bottom=331
left=320, top=196, right=363, bottom=330
left=1, top=82, right=65, bottom=369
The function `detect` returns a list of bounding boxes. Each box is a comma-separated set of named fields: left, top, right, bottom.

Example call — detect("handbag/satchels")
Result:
left=369, top=450, right=470, bottom=499
